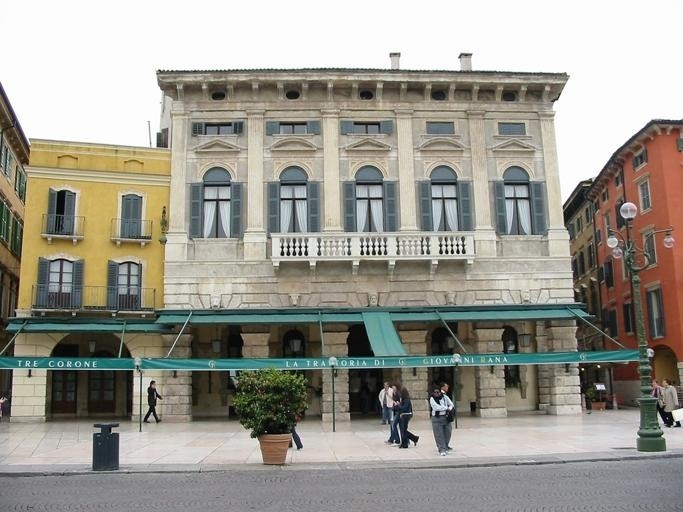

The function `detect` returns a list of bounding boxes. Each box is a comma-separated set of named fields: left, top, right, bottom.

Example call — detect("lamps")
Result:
left=519, top=320, right=532, bottom=346
left=446, top=335, right=457, bottom=349
left=88, top=339, right=96, bottom=353
left=211, top=325, right=222, bottom=353
left=289, top=325, right=301, bottom=353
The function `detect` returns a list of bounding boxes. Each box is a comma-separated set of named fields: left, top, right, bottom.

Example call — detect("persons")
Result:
left=396, top=386, right=421, bottom=451
left=378, top=381, right=392, bottom=424
left=143, top=380, right=163, bottom=423
left=390, top=384, right=399, bottom=406
left=660, top=378, right=679, bottom=426
left=440, top=383, right=449, bottom=394
left=429, top=387, right=456, bottom=457
left=289, top=419, right=304, bottom=451
left=384, top=386, right=400, bottom=446
left=652, top=379, right=668, bottom=426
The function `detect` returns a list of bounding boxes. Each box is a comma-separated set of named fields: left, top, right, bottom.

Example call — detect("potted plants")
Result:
left=227, top=369, right=310, bottom=466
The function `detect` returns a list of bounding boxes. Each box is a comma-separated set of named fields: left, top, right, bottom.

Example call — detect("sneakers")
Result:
left=440, top=447, right=452, bottom=456
left=384, top=436, right=419, bottom=448
left=143, top=420, right=162, bottom=423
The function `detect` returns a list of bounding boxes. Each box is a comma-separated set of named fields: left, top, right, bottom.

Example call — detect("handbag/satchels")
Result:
left=446, top=407, right=456, bottom=422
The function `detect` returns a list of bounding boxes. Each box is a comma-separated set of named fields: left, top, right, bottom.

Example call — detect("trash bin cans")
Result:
left=92, top=423, right=120, bottom=470
